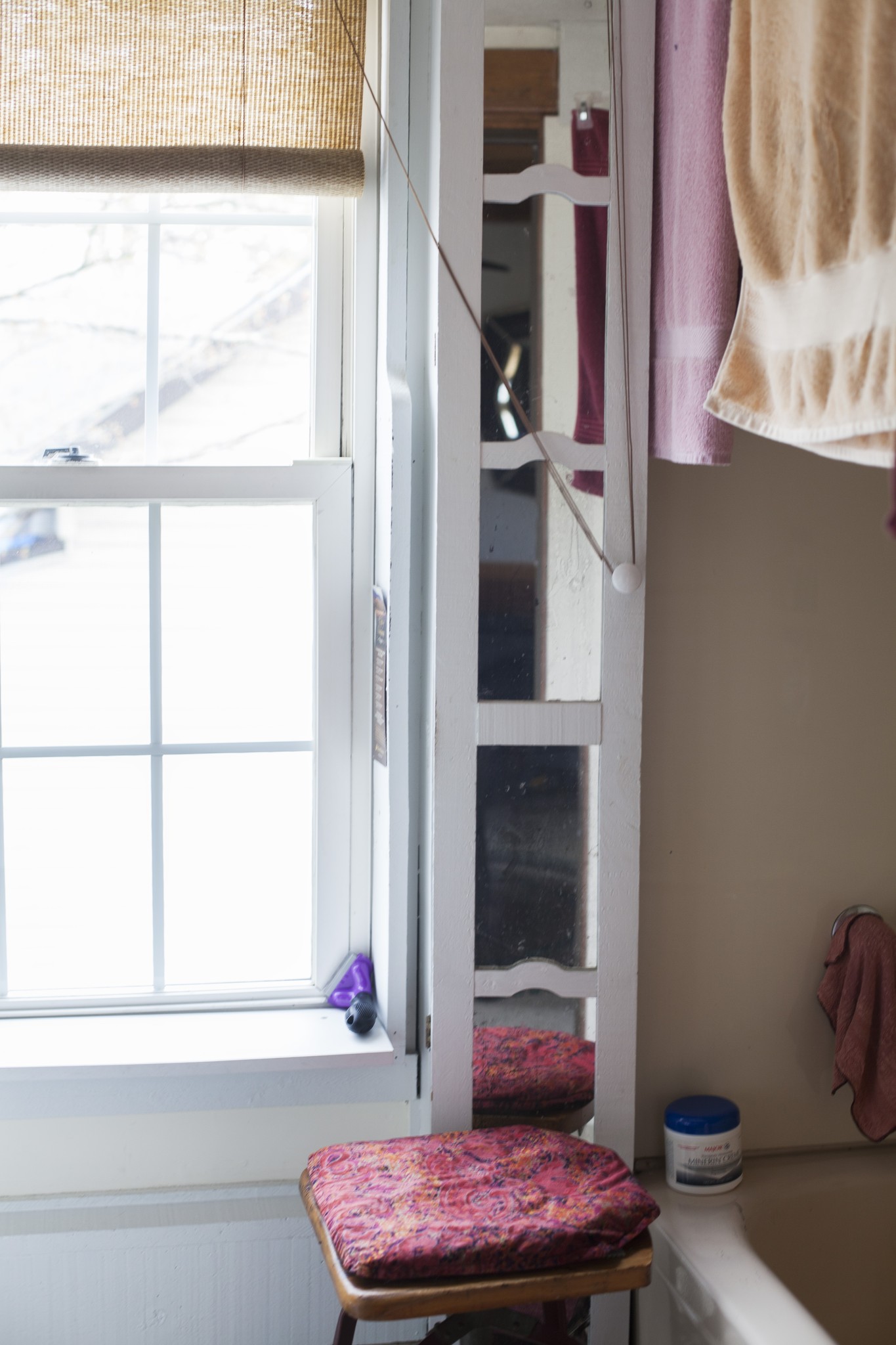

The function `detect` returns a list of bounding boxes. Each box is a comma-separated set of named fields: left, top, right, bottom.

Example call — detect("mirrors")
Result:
left=473, top=4, right=614, bottom=1134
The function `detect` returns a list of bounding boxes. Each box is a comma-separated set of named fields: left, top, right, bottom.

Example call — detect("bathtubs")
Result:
left=635, top=1148, right=896, bottom=1345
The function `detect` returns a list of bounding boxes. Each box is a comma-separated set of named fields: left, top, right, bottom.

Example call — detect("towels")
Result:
left=814, top=905, right=896, bottom=1145
left=644, top=0, right=896, bottom=469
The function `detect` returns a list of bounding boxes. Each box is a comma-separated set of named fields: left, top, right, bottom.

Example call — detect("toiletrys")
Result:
left=661, top=1094, right=744, bottom=1196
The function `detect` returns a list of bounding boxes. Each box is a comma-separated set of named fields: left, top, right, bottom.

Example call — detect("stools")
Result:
left=297, top=1169, right=656, bottom=1345
